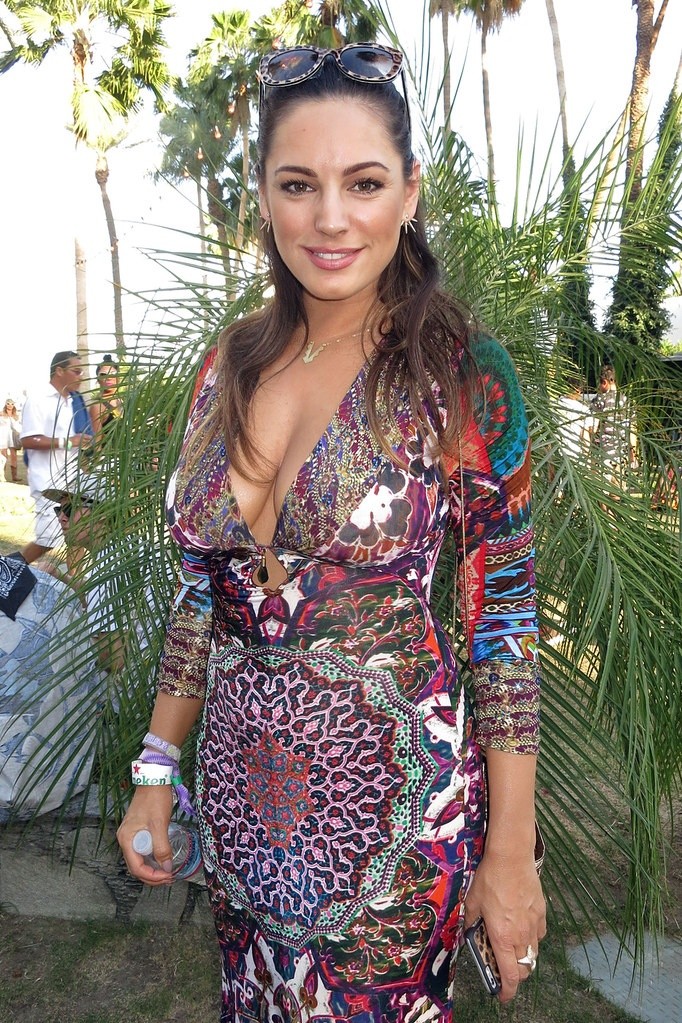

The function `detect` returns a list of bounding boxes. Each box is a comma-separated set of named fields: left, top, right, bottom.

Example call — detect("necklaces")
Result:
left=303, top=326, right=369, bottom=363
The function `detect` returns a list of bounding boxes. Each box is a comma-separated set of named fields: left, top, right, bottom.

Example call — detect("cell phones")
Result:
left=464, top=915, right=502, bottom=995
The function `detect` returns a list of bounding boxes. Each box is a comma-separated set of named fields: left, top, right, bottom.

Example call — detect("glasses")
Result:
left=53, top=503, right=94, bottom=518
left=97, top=372, right=115, bottom=376
left=257, top=41, right=411, bottom=128
left=67, top=369, right=83, bottom=375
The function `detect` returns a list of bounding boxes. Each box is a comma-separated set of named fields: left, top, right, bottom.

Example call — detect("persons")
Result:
left=524, top=359, right=682, bottom=532
left=116, top=41, right=546, bottom=1023
left=0, top=352, right=171, bottom=822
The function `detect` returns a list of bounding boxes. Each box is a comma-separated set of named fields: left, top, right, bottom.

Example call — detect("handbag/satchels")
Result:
left=11, top=428, right=22, bottom=451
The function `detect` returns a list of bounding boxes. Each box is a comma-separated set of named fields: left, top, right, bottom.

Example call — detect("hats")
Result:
left=42, top=473, right=115, bottom=505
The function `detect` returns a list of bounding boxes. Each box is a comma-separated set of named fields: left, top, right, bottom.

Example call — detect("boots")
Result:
left=10, top=466, right=22, bottom=481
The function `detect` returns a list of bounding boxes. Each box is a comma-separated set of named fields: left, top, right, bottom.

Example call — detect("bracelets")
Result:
left=64, top=437, right=72, bottom=451
left=56, top=567, right=63, bottom=579
left=131, top=732, right=196, bottom=816
left=58, top=437, right=64, bottom=450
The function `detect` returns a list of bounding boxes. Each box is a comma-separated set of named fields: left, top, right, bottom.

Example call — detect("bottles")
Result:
left=130, top=822, right=208, bottom=888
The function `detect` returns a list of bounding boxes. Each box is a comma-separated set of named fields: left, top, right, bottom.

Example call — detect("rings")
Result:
left=518, top=945, right=537, bottom=975
